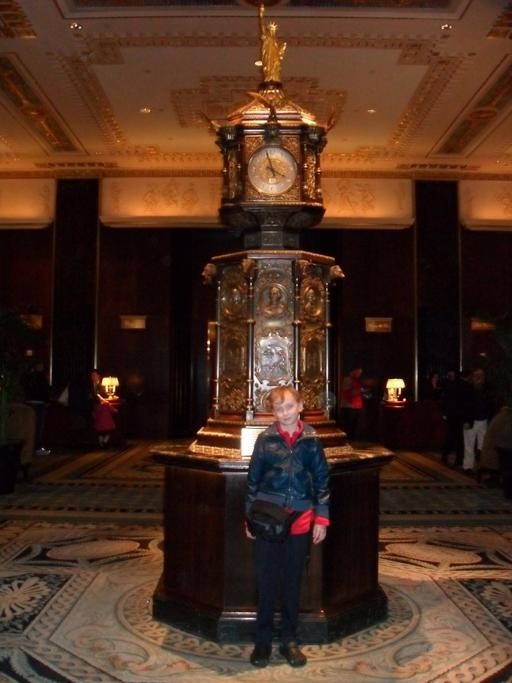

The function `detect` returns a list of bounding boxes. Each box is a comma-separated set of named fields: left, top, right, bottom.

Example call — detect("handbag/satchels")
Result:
left=247, top=499, right=290, bottom=544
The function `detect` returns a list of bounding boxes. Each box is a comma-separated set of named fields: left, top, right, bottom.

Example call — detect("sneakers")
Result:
left=280, top=641, right=307, bottom=667
left=250, top=644, right=272, bottom=667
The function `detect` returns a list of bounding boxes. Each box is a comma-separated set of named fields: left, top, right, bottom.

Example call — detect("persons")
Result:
left=94, top=398, right=119, bottom=448
left=433, top=368, right=465, bottom=470
left=245, top=384, right=332, bottom=668
left=478, top=406, right=511, bottom=505
left=463, top=366, right=490, bottom=468
left=91, top=369, right=101, bottom=386
left=338, top=365, right=365, bottom=409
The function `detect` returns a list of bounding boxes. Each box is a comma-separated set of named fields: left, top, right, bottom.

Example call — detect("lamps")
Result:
left=386, top=377, right=405, bottom=401
left=102, top=377, right=121, bottom=398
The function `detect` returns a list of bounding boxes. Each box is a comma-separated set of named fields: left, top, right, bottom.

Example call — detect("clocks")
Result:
left=247, top=146, right=298, bottom=196
